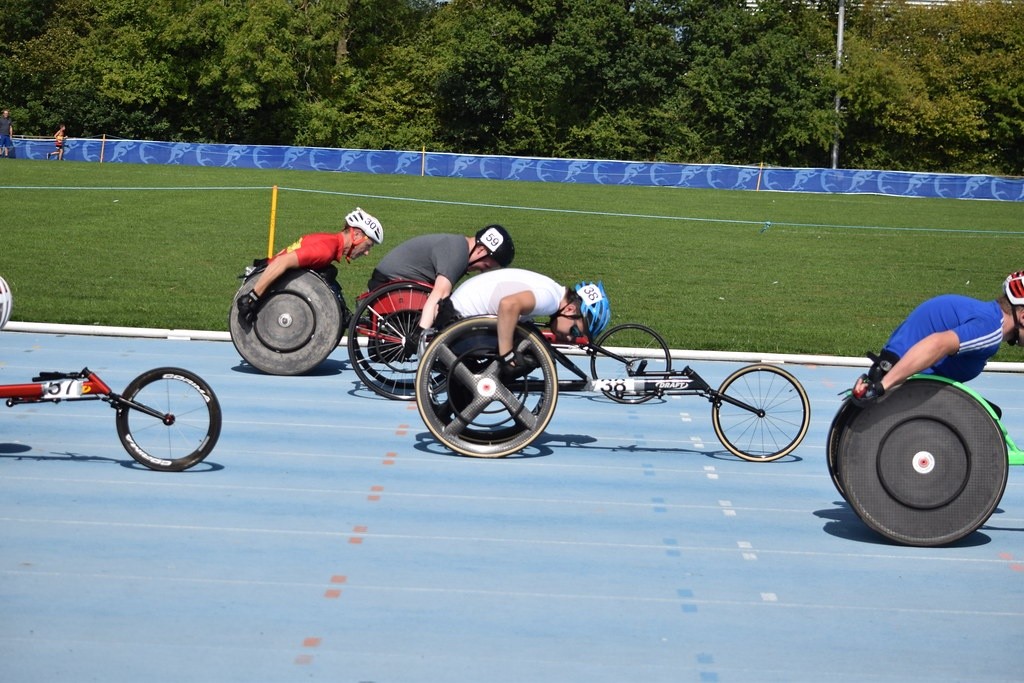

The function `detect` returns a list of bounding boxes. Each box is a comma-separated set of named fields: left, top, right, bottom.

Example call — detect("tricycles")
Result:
left=227, top=269, right=366, bottom=377
left=824, top=374, right=1024, bottom=548
left=0, top=366, right=221, bottom=473
left=414, top=314, right=811, bottom=462
left=346, top=279, right=673, bottom=405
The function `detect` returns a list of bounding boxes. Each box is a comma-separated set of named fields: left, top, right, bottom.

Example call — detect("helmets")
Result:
left=476, top=224, right=515, bottom=267
left=574, top=280, right=611, bottom=342
left=1002, top=270, right=1024, bottom=306
left=345, top=206, right=383, bottom=244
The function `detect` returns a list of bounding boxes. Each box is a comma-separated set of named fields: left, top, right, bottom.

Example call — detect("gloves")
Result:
left=237, top=289, right=261, bottom=323
left=404, top=326, right=426, bottom=358
left=499, top=349, right=536, bottom=382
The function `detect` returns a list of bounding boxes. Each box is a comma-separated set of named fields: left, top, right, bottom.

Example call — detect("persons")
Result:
left=47, top=125, right=66, bottom=160
left=237, top=206, right=383, bottom=327
left=433, top=268, right=611, bottom=379
left=0, top=110, right=13, bottom=158
left=853, top=270, right=1024, bottom=418
left=368, top=225, right=515, bottom=344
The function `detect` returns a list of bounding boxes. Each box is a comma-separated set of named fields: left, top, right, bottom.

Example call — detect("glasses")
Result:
left=569, top=310, right=582, bottom=345
